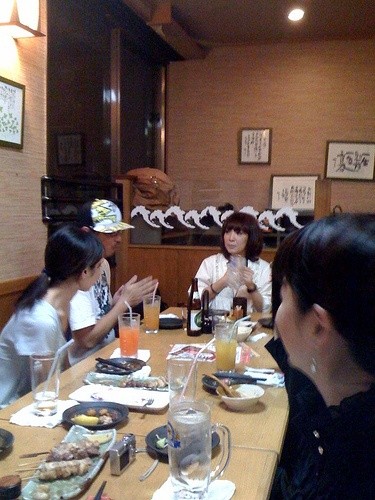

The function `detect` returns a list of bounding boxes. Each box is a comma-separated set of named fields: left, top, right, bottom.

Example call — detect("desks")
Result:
left=0, top=307, right=291, bottom=500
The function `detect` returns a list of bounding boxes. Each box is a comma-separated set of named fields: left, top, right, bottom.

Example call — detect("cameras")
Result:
left=109, top=433, right=136, bottom=476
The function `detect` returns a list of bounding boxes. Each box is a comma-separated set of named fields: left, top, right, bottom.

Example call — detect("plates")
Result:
left=96, top=357, right=145, bottom=375
left=84, top=372, right=169, bottom=391
left=64, top=402, right=129, bottom=429
left=0, top=429, right=14, bottom=456
left=70, top=384, right=169, bottom=413
left=158, top=318, right=182, bottom=329
left=202, top=374, right=257, bottom=390
left=145, top=424, right=220, bottom=456
left=14, top=425, right=116, bottom=500
left=259, top=317, right=274, bottom=328
left=212, top=309, right=229, bottom=316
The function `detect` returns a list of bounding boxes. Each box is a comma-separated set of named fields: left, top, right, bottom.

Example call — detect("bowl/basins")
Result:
left=215, top=384, right=265, bottom=411
left=212, top=314, right=257, bottom=342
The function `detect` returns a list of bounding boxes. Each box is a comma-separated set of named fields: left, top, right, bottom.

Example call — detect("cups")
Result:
left=230, top=287, right=249, bottom=321
left=143, top=294, right=160, bottom=334
left=181, top=300, right=190, bottom=330
left=117, top=312, right=141, bottom=359
left=31, top=352, right=63, bottom=416
left=167, top=357, right=231, bottom=498
left=214, top=324, right=237, bottom=374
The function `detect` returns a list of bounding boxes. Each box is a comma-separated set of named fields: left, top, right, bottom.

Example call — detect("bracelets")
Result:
left=211, top=284, right=219, bottom=294
left=247, top=283, right=257, bottom=293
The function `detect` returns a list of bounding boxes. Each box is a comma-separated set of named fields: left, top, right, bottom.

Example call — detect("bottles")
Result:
left=186, top=278, right=211, bottom=336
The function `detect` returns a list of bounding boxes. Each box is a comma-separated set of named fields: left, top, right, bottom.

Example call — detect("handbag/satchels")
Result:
left=126, top=167, right=180, bottom=215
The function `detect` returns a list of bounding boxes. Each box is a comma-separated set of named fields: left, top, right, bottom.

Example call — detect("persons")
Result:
left=187, top=212, right=273, bottom=313
left=0, top=225, right=103, bottom=409
left=68, top=198, right=158, bottom=367
left=269, top=216, right=375, bottom=500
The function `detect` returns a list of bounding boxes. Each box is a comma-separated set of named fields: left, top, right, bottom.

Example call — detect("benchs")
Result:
left=0, top=275, right=38, bottom=332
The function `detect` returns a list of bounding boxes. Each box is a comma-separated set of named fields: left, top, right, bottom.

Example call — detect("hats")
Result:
left=77, top=199, right=136, bottom=234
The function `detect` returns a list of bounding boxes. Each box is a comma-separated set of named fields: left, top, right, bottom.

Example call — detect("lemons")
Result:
left=86, top=431, right=113, bottom=443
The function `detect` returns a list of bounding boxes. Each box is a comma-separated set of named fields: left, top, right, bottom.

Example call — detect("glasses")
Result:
left=93, top=230, right=125, bottom=236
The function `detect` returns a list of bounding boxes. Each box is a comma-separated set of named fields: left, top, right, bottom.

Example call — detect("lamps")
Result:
left=0, top=0, right=46, bottom=38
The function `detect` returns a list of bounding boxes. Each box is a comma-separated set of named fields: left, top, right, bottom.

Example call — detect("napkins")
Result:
left=150, top=476, right=236, bottom=500
left=10, top=400, right=80, bottom=429
left=243, top=371, right=285, bottom=387
left=159, top=313, right=178, bottom=318
left=250, top=331, right=267, bottom=342
left=110, top=348, right=151, bottom=363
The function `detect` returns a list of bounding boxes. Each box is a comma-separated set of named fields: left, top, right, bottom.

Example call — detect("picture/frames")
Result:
left=269, top=175, right=320, bottom=214
left=238, top=128, right=272, bottom=165
left=324, top=141, right=375, bottom=181
left=54, top=132, right=86, bottom=167
left=0, top=76, right=25, bottom=149
